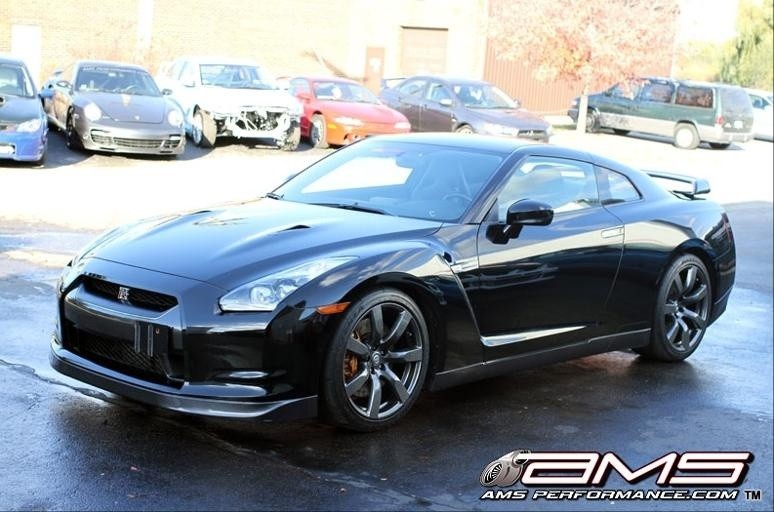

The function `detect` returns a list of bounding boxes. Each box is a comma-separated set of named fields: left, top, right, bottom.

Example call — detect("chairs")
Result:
left=408, top=158, right=472, bottom=205
left=524, top=164, right=564, bottom=216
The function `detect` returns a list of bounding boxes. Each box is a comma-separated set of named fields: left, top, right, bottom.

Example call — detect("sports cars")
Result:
left=49, top=132, right=737, bottom=429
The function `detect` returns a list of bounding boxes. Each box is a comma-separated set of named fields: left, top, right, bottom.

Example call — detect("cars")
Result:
left=742, top=88, right=773, bottom=143
left=0, top=57, right=52, bottom=168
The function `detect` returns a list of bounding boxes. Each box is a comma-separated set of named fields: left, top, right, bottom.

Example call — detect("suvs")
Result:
left=568, top=76, right=752, bottom=152
